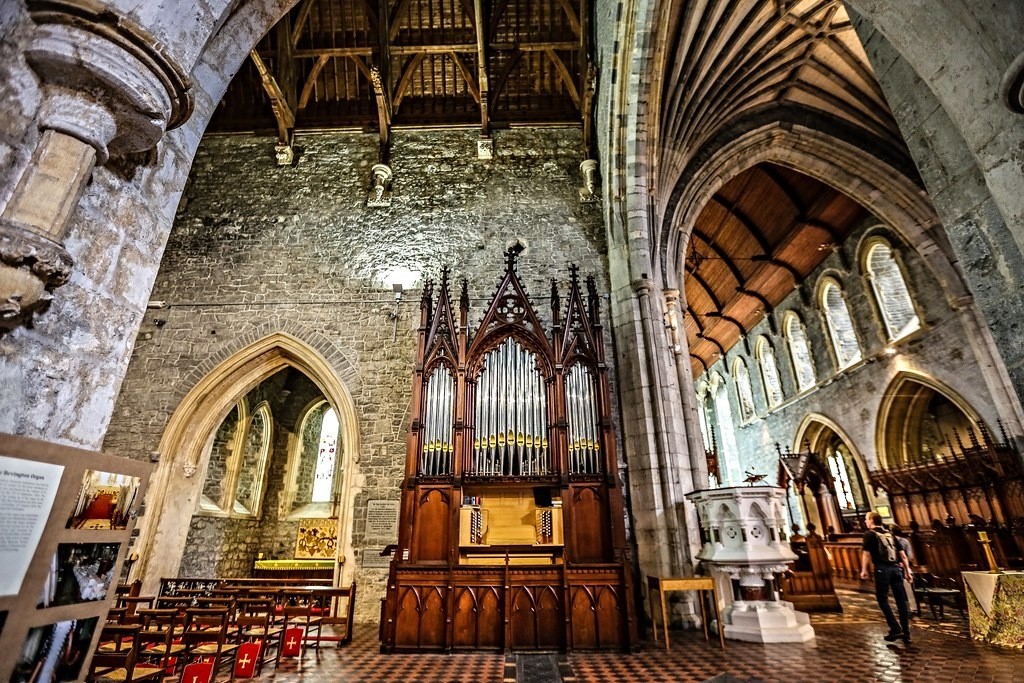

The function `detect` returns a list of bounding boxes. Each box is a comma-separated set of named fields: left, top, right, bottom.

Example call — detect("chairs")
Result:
left=911, top=573, right=966, bottom=625
left=86, top=579, right=324, bottom=683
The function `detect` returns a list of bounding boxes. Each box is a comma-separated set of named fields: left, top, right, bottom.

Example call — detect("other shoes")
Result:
left=884, top=631, right=903, bottom=642
left=902, top=633, right=911, bottom=640
left=908, top=610, right=922, bottom=617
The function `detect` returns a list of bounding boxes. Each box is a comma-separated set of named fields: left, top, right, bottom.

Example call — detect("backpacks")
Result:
left=869, top=529, right=900, bottom=565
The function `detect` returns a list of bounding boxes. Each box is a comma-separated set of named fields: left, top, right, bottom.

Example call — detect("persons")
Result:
left=860, top=511, right=914, bottom=642
left=886, top=523, right=918, bottom=619
left=790, top=523, right=832, bottom=572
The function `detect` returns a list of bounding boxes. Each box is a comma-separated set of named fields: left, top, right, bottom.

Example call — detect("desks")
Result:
left=646, top=574, right=726, bottom=655
left=215, top=579, right=356, bottom=648
left=254, top=559, right=335, bottom=585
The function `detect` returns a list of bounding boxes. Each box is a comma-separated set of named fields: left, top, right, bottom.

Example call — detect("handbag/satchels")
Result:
left=883, top=568, right=904, bottom=585
